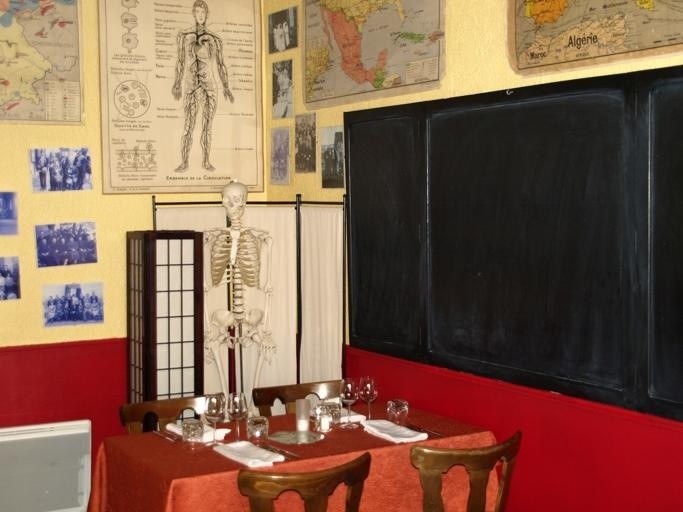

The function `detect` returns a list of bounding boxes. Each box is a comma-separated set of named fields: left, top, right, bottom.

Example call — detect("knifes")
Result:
left=266, top=443, right=299, bottom=457
left=152, top=429, right=175, bottom=443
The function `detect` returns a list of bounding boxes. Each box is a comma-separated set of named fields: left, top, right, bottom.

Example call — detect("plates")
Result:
left=269, top=430, right=324, bottom=444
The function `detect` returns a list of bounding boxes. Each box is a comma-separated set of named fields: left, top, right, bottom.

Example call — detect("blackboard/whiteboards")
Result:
left=344, top=65, right=683, bottom=421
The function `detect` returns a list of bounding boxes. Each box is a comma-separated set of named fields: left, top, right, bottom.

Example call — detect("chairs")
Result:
left=116, top=391, right=226, bottom=437
left=407, top=429, right=523, bottom=510
left=250, top=377, right=344, bottom=420
left=236, top=449, right=371, bottom=512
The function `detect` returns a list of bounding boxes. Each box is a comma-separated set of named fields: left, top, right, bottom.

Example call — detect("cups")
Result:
left=314, top=403, right=341, bottom=433
left=181, top=419, right=203, bottom=452
left=246, top=416, right=269, bottom=450
left=386, top=400, right=409, bottom=426
left=294, top=399, right=310, bottom=432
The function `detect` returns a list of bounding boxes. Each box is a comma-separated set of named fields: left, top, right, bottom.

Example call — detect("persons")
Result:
left=276, top=66, right=290, bottom=93
left=271, top=121, right=343, bottom=182
left=270, top=18, right=290, bottom=52
left=1, top=145, right=102, bottom=324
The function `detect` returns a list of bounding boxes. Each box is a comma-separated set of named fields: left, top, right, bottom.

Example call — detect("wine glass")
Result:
left=204, top=394, right=225, bottom=447
left=226, top=392, right=247, bottom=441
left=358, top=375, right=378, bottom=420
left=339, top=378, right=359, bottom=428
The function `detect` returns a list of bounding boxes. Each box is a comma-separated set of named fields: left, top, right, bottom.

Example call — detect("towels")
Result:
left=163, top=419, right=233, bottom=445
left=211, top=439, right=284, bottom=472
left=307, top=406, right=367, bottom=422
left=357, top=416, right=428, bottom=444
